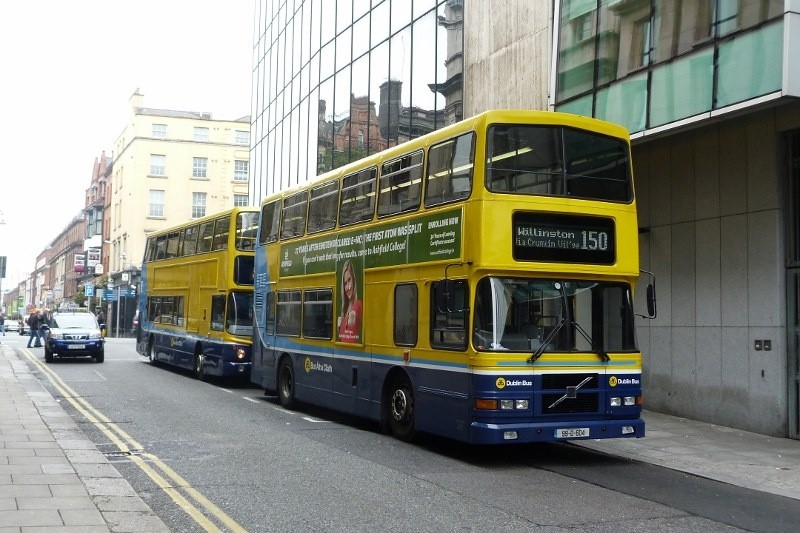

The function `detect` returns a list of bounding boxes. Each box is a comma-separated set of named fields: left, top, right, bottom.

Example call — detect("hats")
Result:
left=36, top=310, right=42, bottom=314
left=44, top=308, right=50, bottom=311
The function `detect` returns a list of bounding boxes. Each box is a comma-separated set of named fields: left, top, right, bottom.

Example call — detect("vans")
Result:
left=19, top=316, right=31, bottom=335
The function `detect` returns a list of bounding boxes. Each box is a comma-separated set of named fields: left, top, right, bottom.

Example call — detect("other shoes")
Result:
left=27, top=346, right=33, bottom=348
left=34, top=345, right=43, bottom=347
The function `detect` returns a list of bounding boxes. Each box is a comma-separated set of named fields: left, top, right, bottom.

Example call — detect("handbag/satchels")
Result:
left=27, top=318, right=31, bottom=326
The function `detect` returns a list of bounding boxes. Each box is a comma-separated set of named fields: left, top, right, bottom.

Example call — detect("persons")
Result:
left=0, top=312, right=5, bottom=336
left=95, top=306, right=108, bottom=337
left=27, top=308, right=50, bottom=348
left=338, top=260, right=363, bottom=344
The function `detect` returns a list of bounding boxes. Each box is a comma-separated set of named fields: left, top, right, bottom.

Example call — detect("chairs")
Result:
left=271, top=188, right=457, bottom=240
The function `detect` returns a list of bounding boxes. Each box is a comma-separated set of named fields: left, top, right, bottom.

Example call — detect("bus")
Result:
left=252, top=108, right=657, bottom=447
left=136, top=205, right=261, bottom=380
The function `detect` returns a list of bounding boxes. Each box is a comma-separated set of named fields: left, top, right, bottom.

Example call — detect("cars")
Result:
left=149, top=314, right=184, bottom=361
left=40, top=310, right=107, bottom=363
left=5, top=320, right=19, bottom=332
left=131, top=309, right=139, bottom=334
